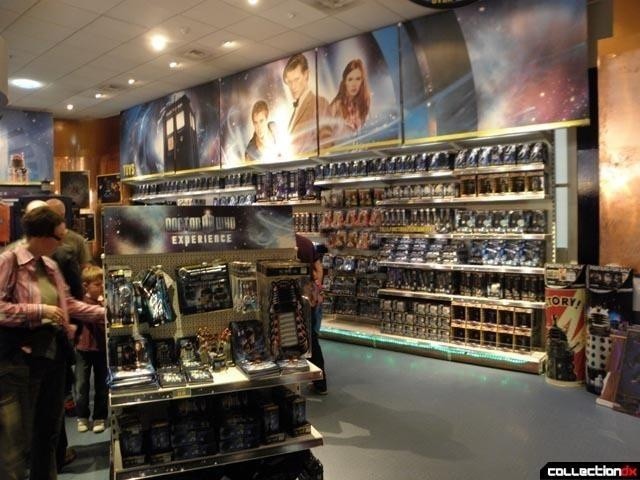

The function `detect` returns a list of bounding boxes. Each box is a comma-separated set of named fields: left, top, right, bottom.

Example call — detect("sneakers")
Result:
left=306, top=383, right=328, bottom=395
left=64, top=448, right=77, bottom=464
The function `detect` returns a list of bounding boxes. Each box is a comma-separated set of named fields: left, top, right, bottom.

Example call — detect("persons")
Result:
left=244, top=52, right=371, bottom=160
left=1, top=197, right=109, bottom=480
left=295, top=232, right=327, bottom=395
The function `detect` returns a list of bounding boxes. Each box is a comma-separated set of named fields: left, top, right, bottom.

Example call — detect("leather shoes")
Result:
left=77, top=418, right=106, bottom=433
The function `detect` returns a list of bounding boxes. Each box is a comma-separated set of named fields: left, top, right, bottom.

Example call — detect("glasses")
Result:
left=52, top=232, right=61, bottom=241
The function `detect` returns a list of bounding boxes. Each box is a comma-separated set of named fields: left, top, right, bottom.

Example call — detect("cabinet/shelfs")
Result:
left=96, top=198, right=332, bottom=480
left=117, top=137, right=559, bottom=377
